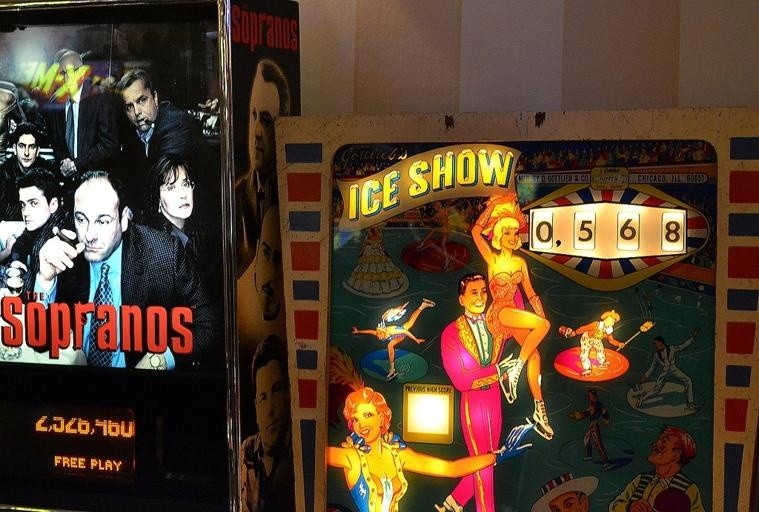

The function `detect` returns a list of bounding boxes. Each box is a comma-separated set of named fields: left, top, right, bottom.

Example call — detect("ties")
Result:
left=87, top=263, right=114, bottom=366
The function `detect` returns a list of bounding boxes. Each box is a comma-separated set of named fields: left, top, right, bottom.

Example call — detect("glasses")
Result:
left=159, top=181, right=197, bottom=192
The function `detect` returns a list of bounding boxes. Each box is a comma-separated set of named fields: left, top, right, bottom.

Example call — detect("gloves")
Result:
left=489, top=424, right=536, bottom=468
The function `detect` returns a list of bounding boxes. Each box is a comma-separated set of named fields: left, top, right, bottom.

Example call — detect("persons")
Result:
left=232, top=201, right=286, bottom=337
left=1, top=46, right=220, bottom=370
left=231, top=58, right=289, bottom=261
left=235, top=333, right=295, bottom=512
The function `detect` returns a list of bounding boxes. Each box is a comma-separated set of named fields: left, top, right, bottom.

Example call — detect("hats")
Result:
left=529, top=472, right=600, bottom=510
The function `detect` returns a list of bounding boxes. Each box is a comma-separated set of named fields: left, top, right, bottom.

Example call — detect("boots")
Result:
left=532, top=398, right=554, bottom=435
left=506, top=355, right=526, bottom=402
